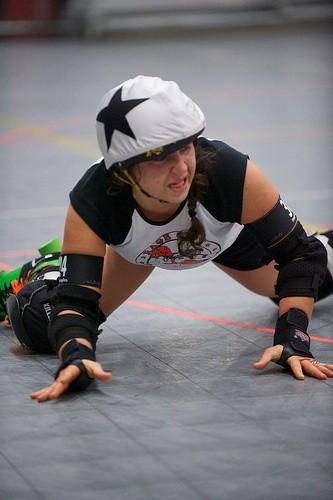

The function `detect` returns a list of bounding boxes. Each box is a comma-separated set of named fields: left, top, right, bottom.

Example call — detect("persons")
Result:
left=0, top=76, right=333, bottom=403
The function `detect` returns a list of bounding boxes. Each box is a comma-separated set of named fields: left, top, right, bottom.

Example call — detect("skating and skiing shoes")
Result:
left=0, top=237, right=65, bottom=327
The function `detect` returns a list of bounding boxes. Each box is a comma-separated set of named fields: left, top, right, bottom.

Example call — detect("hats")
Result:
left=95, top=75, right=205, bottom=171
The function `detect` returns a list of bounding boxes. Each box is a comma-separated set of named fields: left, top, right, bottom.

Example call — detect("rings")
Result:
left=311, top=360, right=320, bottom=366
